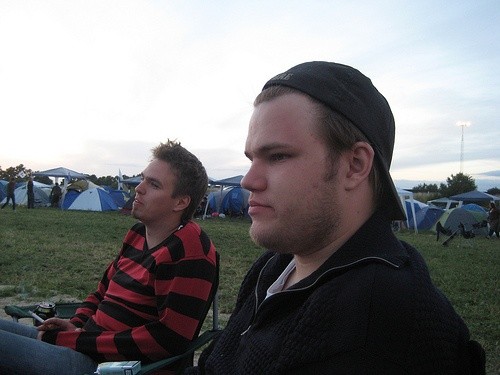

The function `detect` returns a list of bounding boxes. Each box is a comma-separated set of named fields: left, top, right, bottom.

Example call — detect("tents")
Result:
left=216, top=175, right=244, bottom=200
left=120, top=175, right=142, bottom=184
left=396, top=187, right=500, bottom=235
left=0, top=180, right=126, bottom=212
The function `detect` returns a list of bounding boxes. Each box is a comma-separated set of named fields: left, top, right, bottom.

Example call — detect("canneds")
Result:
left=33, top=301, right=55, bottom=326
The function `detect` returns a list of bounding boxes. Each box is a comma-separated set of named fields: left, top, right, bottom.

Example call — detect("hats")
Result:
left=262, top=61, right=408, bottom=222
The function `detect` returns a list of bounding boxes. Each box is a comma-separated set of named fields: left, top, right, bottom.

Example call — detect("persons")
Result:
left=487, top=202, right=500, bottom=239
left=0, top=142, right=219, bottom=375
left=1, top=177, right=16, bottom=211
left=185, top=61, right=487, bottom=375
left=27, top=178, right=34, bottom=209
left=49, top=182, right=61, bottom=208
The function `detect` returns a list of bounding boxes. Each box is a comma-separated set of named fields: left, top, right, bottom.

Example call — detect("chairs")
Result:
left=4, top=251, right=224, bottom=375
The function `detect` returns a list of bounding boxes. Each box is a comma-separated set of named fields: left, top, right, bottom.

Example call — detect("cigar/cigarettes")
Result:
left=29, top=310, right=53, bottom=329
